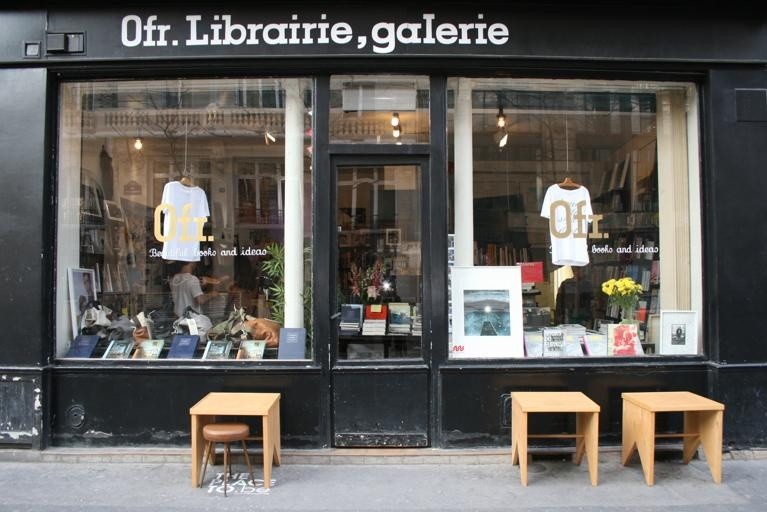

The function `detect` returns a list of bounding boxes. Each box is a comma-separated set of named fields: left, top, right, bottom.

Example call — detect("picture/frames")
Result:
left=658, top=310, right=698, bottom=357
left=385, top=228, right=401, bottom=245
left=67, top=267, right=97, bottom=340
left=636, top=138, right=656, bottom=183
left=80, top=183, right=124, bottom=224
left=595, top=153, right=630, bottom=198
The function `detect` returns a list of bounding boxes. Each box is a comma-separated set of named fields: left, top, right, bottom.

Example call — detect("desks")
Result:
left=620, top=390, right=725, bottom=486
left=339, top=335, right=420, bottom=358
left=509, top=390, right=601, bottom=488
left=188, top=390, right=281, bottom=489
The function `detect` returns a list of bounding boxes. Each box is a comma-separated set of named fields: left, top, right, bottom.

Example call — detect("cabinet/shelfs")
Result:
left=80, top=213, right=135, bottom=320
left=578, top=187, right=660, bottom=342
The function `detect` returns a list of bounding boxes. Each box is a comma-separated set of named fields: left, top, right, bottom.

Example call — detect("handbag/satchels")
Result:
left=209, top=307, right=282, bottom=347
left=172, top=305, right=214, bottom=345
left=81, top=300, right=172, bottom=346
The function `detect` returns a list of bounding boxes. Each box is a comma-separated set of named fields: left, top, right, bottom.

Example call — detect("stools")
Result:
left=199, top=422, right=255, bottom=497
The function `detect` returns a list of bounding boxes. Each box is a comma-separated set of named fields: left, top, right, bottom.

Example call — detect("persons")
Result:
left=77, top=272, right=94, bottom=313
left=169, top=258, right=217, bottom=318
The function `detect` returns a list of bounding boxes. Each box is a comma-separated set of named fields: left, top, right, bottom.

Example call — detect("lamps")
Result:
left=390, top=113, right=401, bottom=138
left=133, top=127, right=143, bottom=150
left=496, top=105, right=506, bottom=129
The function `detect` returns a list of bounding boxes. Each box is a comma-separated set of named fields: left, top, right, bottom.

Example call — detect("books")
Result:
left=471, top=149, right=662, bottom=356
left=277, top=327, right=306, bottom=359
left=131, top=339, right=165, bottom=358
left=200, top=340, right=231, bottom=359
left=99, top=338, right=134, bottom=359
left=234, top=338, right=266, bottom=359
left=79, top=184, right=139, bottom=318
left=166, top=334, right=200, bottom=358
left=66, top=334, right=99, bottom=358
left=336, top=299, right=422, bottom=337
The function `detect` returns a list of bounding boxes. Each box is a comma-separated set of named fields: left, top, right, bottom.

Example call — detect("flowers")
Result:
left=349, top=262, right=394, bottom=304
